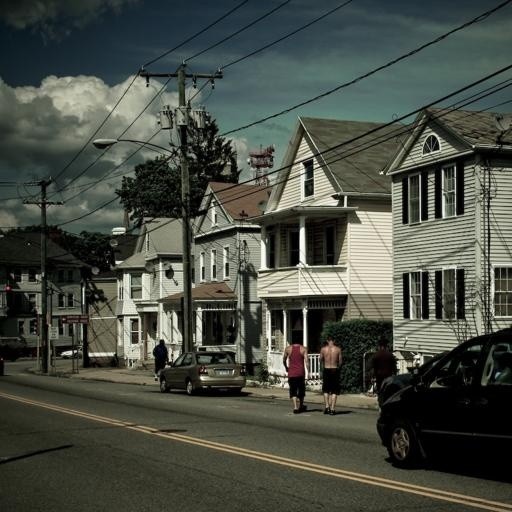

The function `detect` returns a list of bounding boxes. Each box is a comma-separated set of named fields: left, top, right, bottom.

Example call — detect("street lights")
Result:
left=92, top=138, right=195, bottom=357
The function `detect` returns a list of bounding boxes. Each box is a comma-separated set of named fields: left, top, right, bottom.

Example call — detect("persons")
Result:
left=319, top=336, right=344, bottom=415
left=366, top=340, right=397, bottom=409
left=152, top=340, right=169, bottom=381
left=282, top=338, right=311, bottom=413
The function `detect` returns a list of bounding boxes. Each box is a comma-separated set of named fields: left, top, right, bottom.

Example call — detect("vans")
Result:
left=374, top=328, right=512, bottom=483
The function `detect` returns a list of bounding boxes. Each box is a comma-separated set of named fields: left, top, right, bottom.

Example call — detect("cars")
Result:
left=60, top=345, right=83, bottom=359
left=377, top=351, right=481, bottom=412
left=159, top=347, right=246, bottom=396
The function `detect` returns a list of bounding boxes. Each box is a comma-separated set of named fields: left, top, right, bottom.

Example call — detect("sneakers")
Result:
left=293, top=405, right=307, bottom=414
left=323, top=407, right=336, bottom=415
